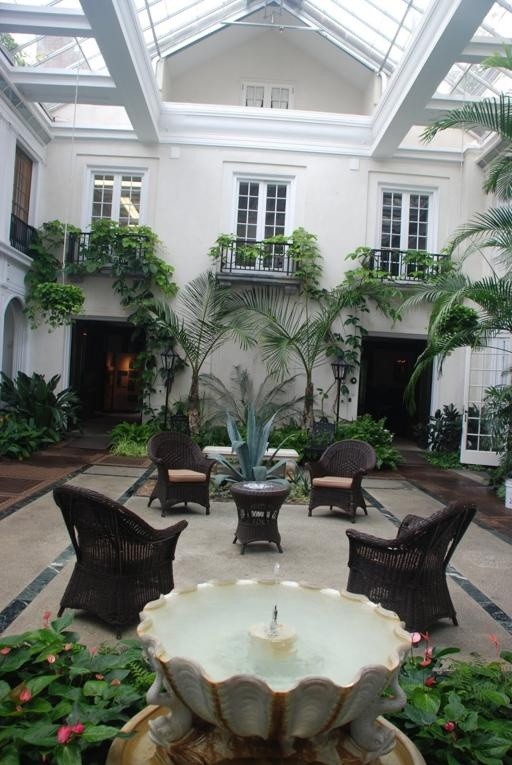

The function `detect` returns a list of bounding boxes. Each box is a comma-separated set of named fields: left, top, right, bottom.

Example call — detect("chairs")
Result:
left=146, top=431, right=217, bottom=519
left=303, top=438, right=376, bottom=523
left=345, top=498, right=477, bottom=648
left=51, top=485, right=187, bottom=638
